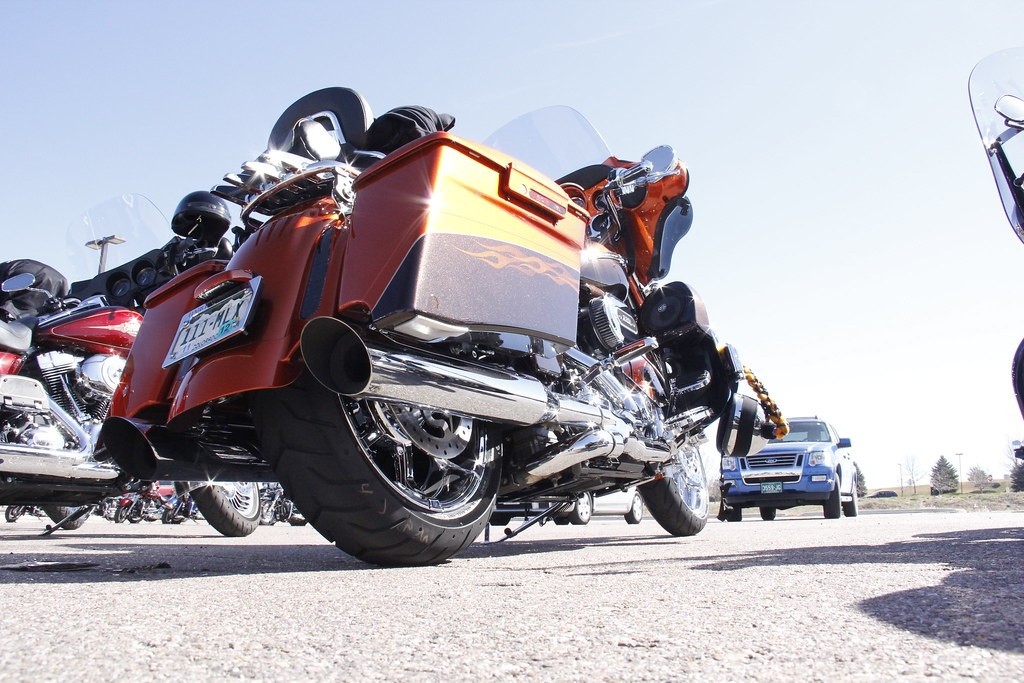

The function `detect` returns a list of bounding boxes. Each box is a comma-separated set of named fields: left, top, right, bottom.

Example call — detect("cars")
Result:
left=489, top=483, right=644, bottom=527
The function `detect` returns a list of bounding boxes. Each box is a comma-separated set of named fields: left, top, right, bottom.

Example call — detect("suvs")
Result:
left=720, top=417, right=860, bottom=523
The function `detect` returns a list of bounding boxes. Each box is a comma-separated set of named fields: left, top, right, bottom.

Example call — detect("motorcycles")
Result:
left=963, top=37, right=1024, bottom=446
left=0, top=258, right=309, bottom=536
left=97, top=86, right=791, bottom=569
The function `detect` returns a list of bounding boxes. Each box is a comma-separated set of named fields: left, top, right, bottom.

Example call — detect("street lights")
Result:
left=88, top=236, right=123, bottom=276
